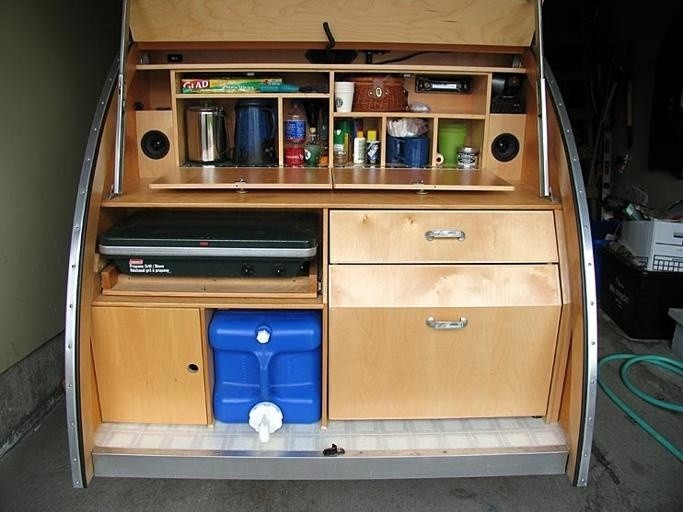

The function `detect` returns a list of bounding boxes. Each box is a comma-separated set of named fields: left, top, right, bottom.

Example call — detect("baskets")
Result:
left=353, top=77, right=409, bottom=112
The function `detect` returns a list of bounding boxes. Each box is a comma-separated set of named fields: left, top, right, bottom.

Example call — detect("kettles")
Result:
left=232, top=100, right=278, bottom=167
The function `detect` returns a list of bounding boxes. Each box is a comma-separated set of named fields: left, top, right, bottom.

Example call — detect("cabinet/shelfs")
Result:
left=65, top=0, right=598, bottom=490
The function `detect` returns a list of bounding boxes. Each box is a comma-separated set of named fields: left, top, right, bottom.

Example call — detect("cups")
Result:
left=438, top=122, right=468, bottom=168
left=335, top=82, right=354, bottom=112
left=185, top=101, right=235, bottom=164
left=387, top=137, right=430, bottom=168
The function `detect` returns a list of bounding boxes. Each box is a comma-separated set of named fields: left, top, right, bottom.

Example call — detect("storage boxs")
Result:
left=620, top=220, right=682, bottom=273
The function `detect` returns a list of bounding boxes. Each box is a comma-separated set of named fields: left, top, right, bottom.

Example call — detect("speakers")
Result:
left=135, top=110, right=185, bottom=182
left=484, top=111, right=526, bottom=184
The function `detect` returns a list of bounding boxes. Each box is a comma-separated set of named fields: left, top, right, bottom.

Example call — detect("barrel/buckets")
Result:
left=207, top=308, right=322, bottom=441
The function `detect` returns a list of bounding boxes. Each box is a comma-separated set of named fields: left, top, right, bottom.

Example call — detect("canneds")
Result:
left=284, top=119, right=305, bottom=144
left=365, top=139, right=379, bottom=164
left=283, top=145, right=305, bottom=166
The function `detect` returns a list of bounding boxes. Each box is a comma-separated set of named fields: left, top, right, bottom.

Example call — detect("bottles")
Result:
left=456, top=147, right=480, bottom=170
left=609, top=240, right=632, bottom=259
left=283, top=103, right=380, bottom=167
